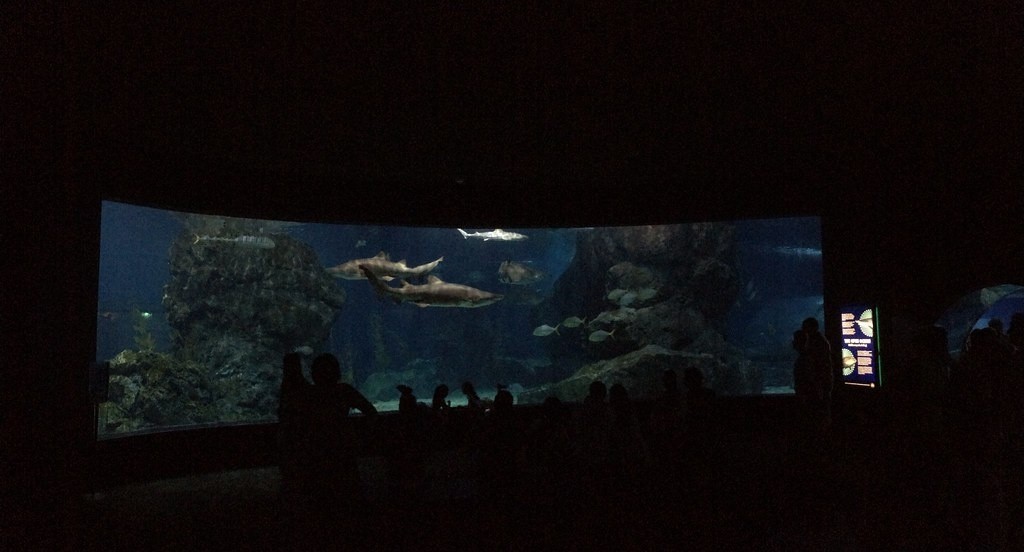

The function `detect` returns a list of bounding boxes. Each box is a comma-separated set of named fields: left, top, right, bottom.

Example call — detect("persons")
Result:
left=270, top=317, right=1024, bottom=552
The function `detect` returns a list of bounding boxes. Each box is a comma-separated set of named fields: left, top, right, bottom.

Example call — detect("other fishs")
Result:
left=192, top=230, right=275, bottom=250
left=532, top=286, right=661, bottom=342
left=294, top=346, right=314, bottom=355
